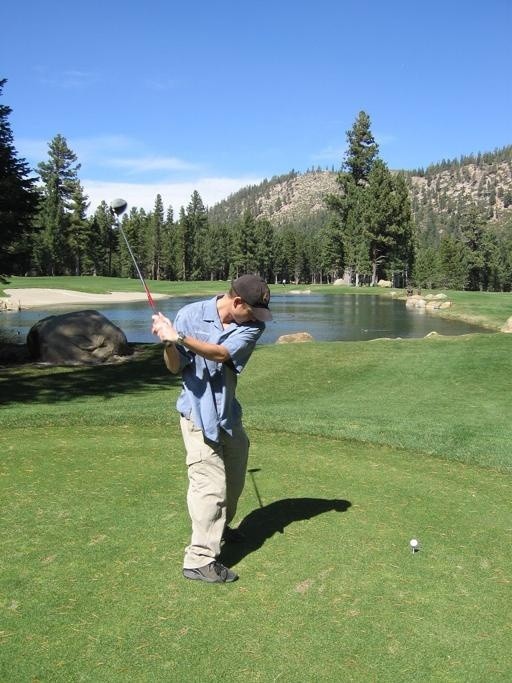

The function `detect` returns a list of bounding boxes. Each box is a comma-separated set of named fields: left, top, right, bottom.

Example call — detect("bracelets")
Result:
left=177, top=330, right=185, bottom=344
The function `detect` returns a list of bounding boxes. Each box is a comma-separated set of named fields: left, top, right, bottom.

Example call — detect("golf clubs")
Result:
left=113, top=198, right=173, bottom=346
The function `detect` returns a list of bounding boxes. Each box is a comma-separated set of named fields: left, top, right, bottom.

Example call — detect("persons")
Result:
left=149, top=272, right=275, bottom=586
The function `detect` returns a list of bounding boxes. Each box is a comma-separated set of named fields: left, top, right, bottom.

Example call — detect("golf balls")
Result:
left=410, top=539, right=417, bottom=547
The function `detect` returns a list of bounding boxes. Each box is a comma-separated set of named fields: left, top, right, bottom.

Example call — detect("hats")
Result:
left=232, top=275, right=272, bottom=322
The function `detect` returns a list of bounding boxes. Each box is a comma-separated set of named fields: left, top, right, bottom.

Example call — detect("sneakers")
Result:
left=182, top=561, right=239, bottom=583
left=222, top=526, right=242, bottom=541
